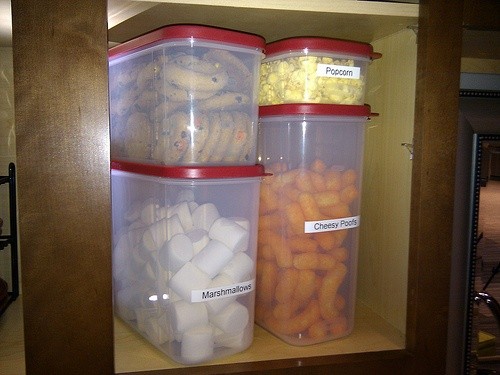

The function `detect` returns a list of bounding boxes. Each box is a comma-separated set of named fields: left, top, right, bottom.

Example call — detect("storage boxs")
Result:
left=107, top=25, right=268, bottom=168
left=256, top=103, right=382, bottom=347
left=260, top=37, right=384, bottom=105
left=110, top=159, right=275, bottom=366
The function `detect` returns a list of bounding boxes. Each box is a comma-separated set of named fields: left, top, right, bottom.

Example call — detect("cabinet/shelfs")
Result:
left=11, top=0, right=465, bottom=375
left=1, top=162, right=20, bottom=315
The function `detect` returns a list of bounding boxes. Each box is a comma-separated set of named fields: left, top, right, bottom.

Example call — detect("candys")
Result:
left=111, top=192, right=251, bottom=362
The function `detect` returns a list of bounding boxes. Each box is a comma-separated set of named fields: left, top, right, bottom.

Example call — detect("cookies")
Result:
left=105, top=51, right=253, bottom=164
